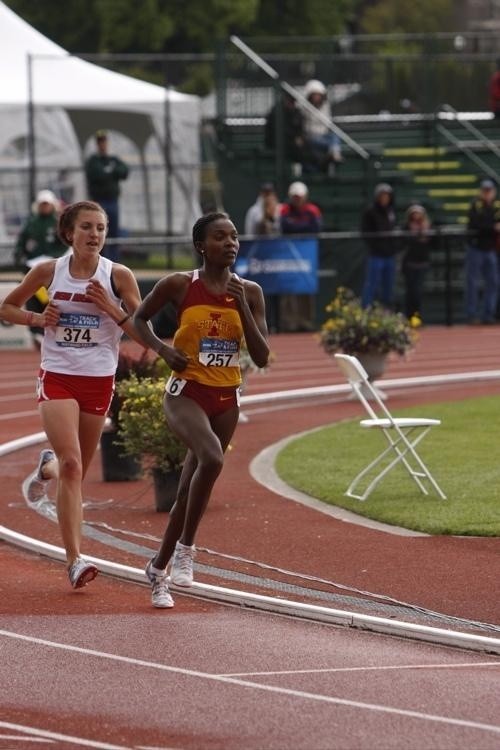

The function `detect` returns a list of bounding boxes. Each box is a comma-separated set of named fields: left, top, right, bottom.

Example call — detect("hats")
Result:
left=288, top=182, right=308, bottom=196
left=37, top=190, right=55, bottom=203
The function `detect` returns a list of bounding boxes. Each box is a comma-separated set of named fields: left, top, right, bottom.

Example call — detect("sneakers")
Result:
left=145, top=540, right=196, bottom=608
left=69, top=557, right=98, bottom=589
left=28, top=449, right=57, bottom=503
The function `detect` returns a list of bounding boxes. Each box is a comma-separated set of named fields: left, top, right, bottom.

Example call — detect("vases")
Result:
left=345, top=354, right=389, bottom=403
left=154, top=467, right=182, bottom=513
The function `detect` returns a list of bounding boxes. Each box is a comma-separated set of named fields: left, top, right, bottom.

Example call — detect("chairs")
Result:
left=333, top=352, right=449, bottom=503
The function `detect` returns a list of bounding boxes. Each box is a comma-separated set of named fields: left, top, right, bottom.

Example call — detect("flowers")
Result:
left=315, top=285, right=424, bottom=360
left=111, top=368, right=189, bottom=470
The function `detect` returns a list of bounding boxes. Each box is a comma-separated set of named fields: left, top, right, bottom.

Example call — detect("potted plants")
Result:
left=94, top=346, right=159, bottom=483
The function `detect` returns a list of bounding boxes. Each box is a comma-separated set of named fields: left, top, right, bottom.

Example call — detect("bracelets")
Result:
left=116, top=311, right=132, bottom=329
left=26, top=311, right=33, bottom=327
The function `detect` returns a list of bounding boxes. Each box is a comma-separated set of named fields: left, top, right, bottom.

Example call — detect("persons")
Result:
left=252, top=194, right=283, bottom=331
left=130, top=212, right=271, bottom=610
left=400, top=197, right=440, bottom=322
left=84, top=137, right=129, bottom=259
left=358, top=181, right=399, bottom=312
left=10, top=188, right=72, bottom=351
left=263, top=88, right=325, bottom=182
left=277, top=182, right=324, bottom=333
left=489, top=63, right=500, bottom=119
left=295, top=78, right=346, bottom=181
left=243, top=183, right=284, bottom=237
left=460, top=178, right=500, bottom=326
left=1, top=200, right=155, bottom=593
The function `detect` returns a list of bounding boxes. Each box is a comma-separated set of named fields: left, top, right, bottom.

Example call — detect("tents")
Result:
left=0, top=1, right=207, bottom=269
